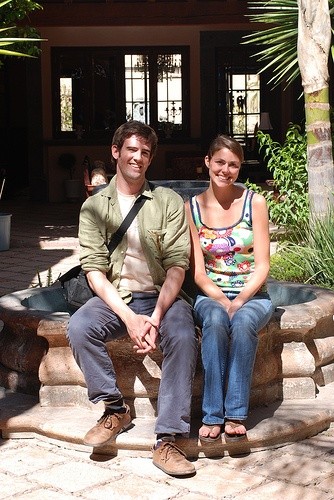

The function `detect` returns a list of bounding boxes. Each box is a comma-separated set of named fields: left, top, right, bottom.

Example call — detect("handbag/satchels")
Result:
left=57, top=264, right=96, bottom=310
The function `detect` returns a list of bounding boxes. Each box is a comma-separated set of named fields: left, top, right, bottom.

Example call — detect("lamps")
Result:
left=259, top=112, right=273, bottom=130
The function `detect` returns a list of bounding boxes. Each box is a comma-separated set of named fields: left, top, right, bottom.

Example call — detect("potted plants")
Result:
left=59, top=152, right=83, bottom=200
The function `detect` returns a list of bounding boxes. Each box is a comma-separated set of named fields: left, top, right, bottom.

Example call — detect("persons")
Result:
left=184, top=134, right=272, bottom=441
left=67, top=121, right=196, bottom=479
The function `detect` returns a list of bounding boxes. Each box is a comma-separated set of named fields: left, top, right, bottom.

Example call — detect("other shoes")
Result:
left=152, top=441, right=197, bottom=477
left=82, top=403, right=133, bottom=448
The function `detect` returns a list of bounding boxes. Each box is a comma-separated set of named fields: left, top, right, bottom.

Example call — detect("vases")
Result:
left=0, top=213, right=12, bottom=251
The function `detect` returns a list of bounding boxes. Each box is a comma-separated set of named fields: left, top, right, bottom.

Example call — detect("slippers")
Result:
left=223, top=420, right=247, bottom=443
left=198, top=424, right=223, bottom=443
